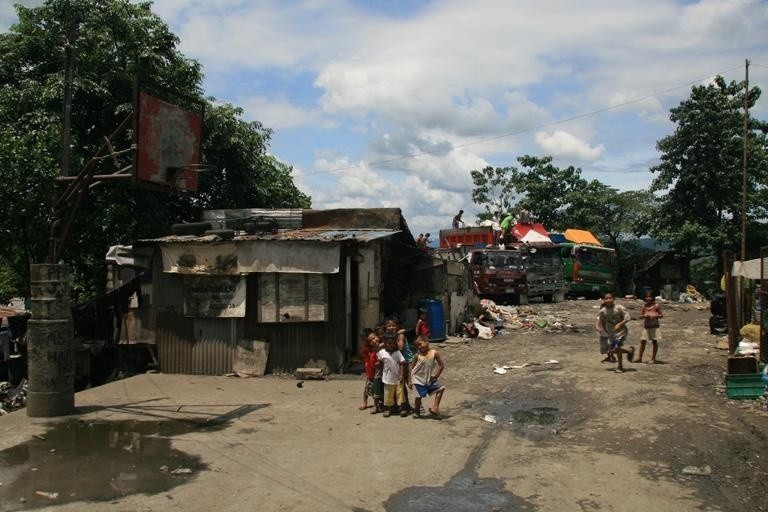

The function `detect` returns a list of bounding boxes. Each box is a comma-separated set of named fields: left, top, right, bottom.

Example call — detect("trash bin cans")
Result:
left=416, top=298, right=446, bottom=343
left=642, top=288, right=653, bottom=300
left=664, top=285, right=680, bottom=302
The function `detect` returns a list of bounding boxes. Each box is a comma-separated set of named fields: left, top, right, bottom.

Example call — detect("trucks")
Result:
left=434, top=222, right=618, bottom=303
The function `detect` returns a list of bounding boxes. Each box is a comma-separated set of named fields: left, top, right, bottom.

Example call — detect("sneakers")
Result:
left=611, top=358, right=616, bottom=362
left=632, top=360, right=642, bottom=363
left=603, top=358, right=610, bottom=361
left=615, top=368, right=623, bottom=373
left=628, top=347, right=634, bottom=361
left=646, top=359, right=655, bottom=363
left=370, top=405, right=443, bottom=420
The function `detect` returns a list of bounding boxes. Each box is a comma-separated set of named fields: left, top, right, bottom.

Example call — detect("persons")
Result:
left=596, top=292, right=635, bottom=372
left=490, top=210, right=502, bottom=240
left=450, top=209, right=464, bottom=230
left=599, top=304, right=616, bottom=363
left=499, top=213, right=516, bottom=240
left=355, top=316, right=446, bottom=419
left=119, top=310, right=142, bottom=344
left=415, top=233, right=423, bottom=248
left=520, top=204, right=531, bottom=223
left=420, top=233, right=432, bottom=250
left=635, top=289, right=663, bottom=364
left=415, top=308, right=431, bottom=339
